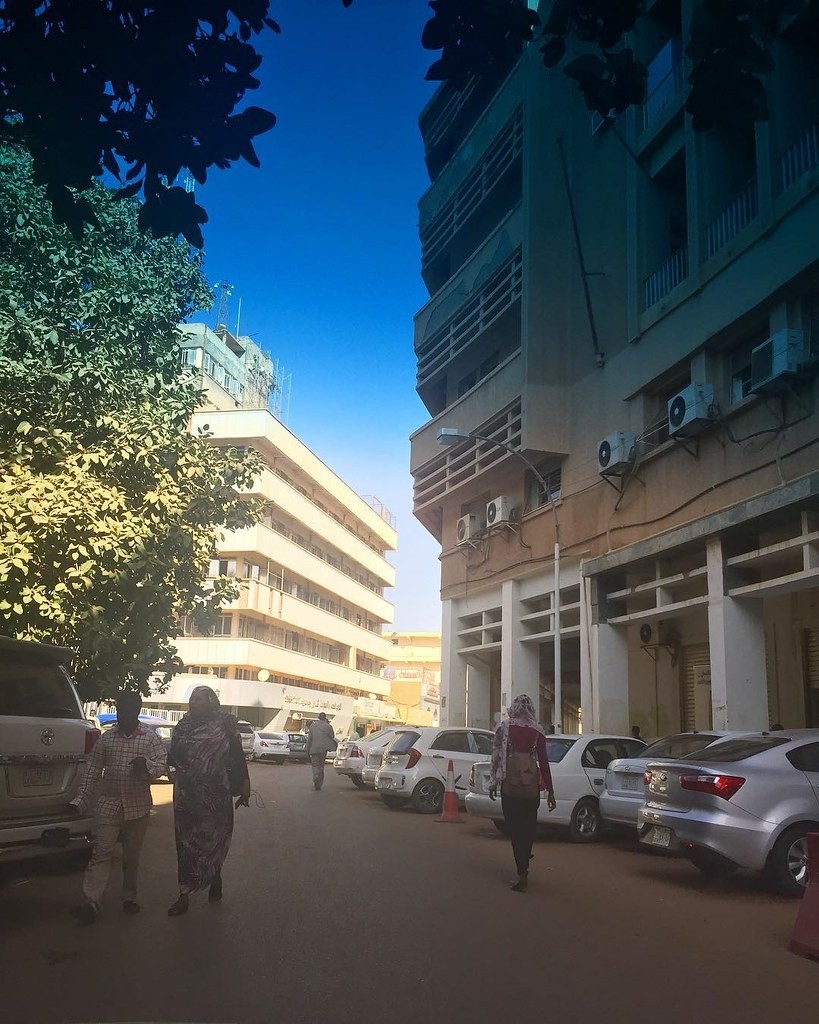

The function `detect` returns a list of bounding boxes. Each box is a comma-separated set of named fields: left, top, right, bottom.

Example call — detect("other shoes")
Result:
left=167, top=894, right=189, bottom=917
left=208, top=878, right=223, bottom=902
left=122, top=900, right=140, bottom=913
left=510, top=883, right=527, bottom=893
left=68, top=901, right=100, bottom=925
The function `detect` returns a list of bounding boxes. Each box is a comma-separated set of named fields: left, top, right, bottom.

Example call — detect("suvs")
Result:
left=373, top=726, right=500, bottom=814
left=237, top=719, right=255, bottom=762
left=0, top=636, right=101, bottom=874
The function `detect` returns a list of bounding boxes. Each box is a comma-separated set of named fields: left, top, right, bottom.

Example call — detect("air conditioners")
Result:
left=638, top=617, right=678, bottom=649
left=596, top=431, right=636, bottom=475
left=455, top=511, right=482, bottom=543
left=747, top=328, right=807, bottom=392
left=485, top=494, right=514, bottom=529
left=666, top=381, right=716, bottom=437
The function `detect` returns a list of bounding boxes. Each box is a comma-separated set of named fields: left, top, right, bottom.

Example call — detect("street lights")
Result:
left=435, top=427, right=562, bottom=736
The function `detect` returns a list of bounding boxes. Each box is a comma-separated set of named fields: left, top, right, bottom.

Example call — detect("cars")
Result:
left=99, top=717, right=179, bottom=784
left=599, top=730, right=743, bottom=852
left=287, top=733, right=310, bottom=764
left=333, top=725, right=393, bottom=789
left=464, top=734, right=651, bottom=844
left=252, top=731, right=290, bottom=766
left=637, top=727, right=819, bottom=902
left=361, top=736, right=390, bottom=787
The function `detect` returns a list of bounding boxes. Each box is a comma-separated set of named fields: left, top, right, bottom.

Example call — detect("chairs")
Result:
left=595, top=749, right=613, bottom=768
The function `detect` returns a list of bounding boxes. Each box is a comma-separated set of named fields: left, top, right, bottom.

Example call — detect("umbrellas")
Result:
left=138, top=715, right=177, bottom=729
left=95, top=714, right=150, bottom=726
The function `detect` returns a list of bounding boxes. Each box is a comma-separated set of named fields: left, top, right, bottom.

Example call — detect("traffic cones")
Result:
left=434, top=760, right=466, bottom=824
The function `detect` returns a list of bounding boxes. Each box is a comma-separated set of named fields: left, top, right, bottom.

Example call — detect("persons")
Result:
left=165, top=685, right=251, bottom=915
left=488, top=694, right=556, bottom=892
left=61, top=690, right=168, bottom=922
left=86, top=709, right=102, bottom=730
left=352, top=727, right=362, bottom=741
left=629, top=726, right=646, bottom=757
left=306, top=719, right=313, bottom=728
left=306, top=712, right=334, bottom=790
left=769, top=724, right=784, bottom=732
left=370, top=729, right=376, bottom=733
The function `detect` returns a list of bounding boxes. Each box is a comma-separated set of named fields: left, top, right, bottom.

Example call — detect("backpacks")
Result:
left=500, top=732, right=539, bottom=800
